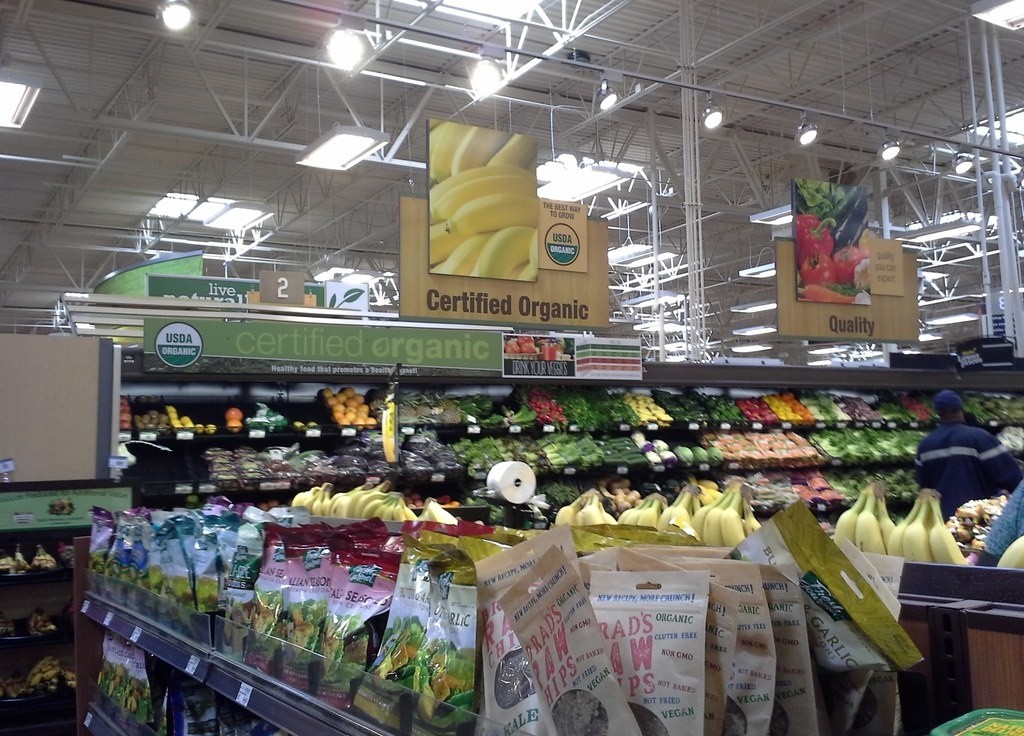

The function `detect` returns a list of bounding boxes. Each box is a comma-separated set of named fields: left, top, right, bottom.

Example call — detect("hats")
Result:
left=934, top=389, right=962, bottom=412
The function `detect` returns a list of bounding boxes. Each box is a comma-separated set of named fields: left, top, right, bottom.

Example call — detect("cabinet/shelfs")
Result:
left=74, top=537, right=538, bottom=736
left=0, top=478, right=138, bottom=736
left=98, top=338, right=1024, bottom=532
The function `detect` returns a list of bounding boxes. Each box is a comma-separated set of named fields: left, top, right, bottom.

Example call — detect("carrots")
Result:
left=803, top=286, right=856, bottom=303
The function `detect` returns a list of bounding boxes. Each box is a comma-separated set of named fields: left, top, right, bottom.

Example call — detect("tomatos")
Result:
left=834, top=245, right=861, bottom=282
left=801, top=251, right=835, bottom=285
left=795, top=215, right=835, bottom=267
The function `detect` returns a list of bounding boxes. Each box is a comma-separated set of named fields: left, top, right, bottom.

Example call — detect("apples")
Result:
left=323, top=387, right=377, bottom=430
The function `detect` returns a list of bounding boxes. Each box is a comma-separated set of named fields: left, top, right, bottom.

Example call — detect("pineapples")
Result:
left=369, top=388, right=461, bottom=424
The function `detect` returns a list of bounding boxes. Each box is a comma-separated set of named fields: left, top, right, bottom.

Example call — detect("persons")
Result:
left=910, top=385, right=1024, bottom=523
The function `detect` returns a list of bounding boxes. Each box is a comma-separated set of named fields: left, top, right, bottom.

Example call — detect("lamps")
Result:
left=295, top=121, right=390, bottom=171
left=877, top=127, right=901, bottom=160
left=895, top=218, right=981, bottom=242
left=204, top=200, right=271, bottom=231
left=750, top=204, right=793, bottom=225
left=739, top=247, right=777, bottom=278
left=322, top=15, right=366, bottom=69
left=596, top=70, right=623, bottom=109
left=618, top=247, right=680, bottom=267
left=0, top=69, right=43, bottom=129
left=701, top=91, right=723, bottom=129
left=951, top=144, right=973, bottom=174
left=970, top=0, right=1024, bottom=30
left=155, top=0, right=195, bottom=33
left=730, top=300, right=777, bottom=313
left=471, top=43, right=505, bottom=94
left=608, top=201, right=652, bottom=264
left=924, top=312, right=978, bottom=325
left=732, top=325, right=777, bottom=335
left=621, top=291, right=679, bottom=307
left=795, top=110, right=818, bottom=145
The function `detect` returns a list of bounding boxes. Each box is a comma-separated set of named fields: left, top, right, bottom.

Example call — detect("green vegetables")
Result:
left=800, top=388, right=988, bottom=503
left=795, top=178, right=849, bottom=220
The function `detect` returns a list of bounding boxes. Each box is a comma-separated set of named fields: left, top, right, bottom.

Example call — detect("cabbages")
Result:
left=691, top=446, right=708, bottom=464
left=707, top=447, right=724, bottom=466
left=674, top=446, right=694, bottom=469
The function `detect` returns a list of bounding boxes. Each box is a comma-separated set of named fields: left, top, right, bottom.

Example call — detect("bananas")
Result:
left=28, top=609, right=56, bottom=637
left=302, top=481, right=1024, bottom=571
left=428, top=119, right=539, bottom=282
left=0, top=653, right=76, bottom=697
left=0, top=612, right=15, bottom=636
left=0, top=542, right=56, bottom=574
